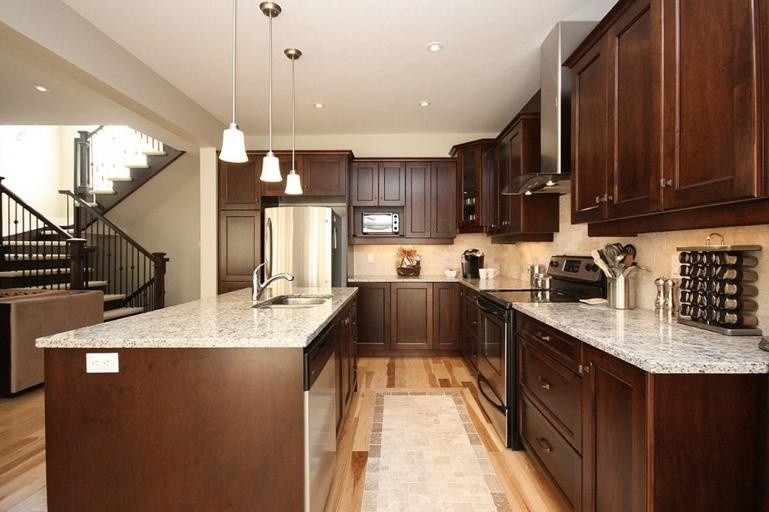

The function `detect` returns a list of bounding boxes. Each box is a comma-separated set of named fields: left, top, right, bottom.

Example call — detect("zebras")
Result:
left=478, top=307, right=516, bottom=449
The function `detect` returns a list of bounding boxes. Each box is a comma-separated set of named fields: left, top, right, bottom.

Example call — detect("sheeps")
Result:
left=249, top=259, right=294, bottom=299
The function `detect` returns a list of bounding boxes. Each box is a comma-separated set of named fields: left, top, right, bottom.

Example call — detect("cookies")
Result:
left=362, top=212, right=400, bottom=235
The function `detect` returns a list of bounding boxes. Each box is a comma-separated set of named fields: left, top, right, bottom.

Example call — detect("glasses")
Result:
left=218, top=0, right=249, bottom=163
left=284, top=48, right=304, bottom=197
left=259, top=1, right=284, bottom=183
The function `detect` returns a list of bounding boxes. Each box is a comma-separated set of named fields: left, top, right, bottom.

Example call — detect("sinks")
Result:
left=0, top=289, right=104, bottom=396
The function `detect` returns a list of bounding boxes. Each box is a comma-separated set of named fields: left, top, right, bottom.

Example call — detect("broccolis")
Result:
left=264, top=207, right=342, bottom=288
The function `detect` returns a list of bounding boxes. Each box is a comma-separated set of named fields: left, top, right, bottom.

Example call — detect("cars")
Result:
left=479, top=269, right=489, bottom=280
left=488, top=268, right=499, bottom=280
left=607, top=277, right=636, bottom=310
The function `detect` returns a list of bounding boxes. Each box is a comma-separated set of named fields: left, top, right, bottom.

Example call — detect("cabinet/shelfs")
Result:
left=653, top=276, right=667, bottom=308
left=664, top=278, right=678, bottom=310
left=530, top=263, right=552, bottom=291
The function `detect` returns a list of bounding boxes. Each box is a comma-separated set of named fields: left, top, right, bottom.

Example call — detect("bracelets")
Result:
left=445, top=270, right=456, bottom=277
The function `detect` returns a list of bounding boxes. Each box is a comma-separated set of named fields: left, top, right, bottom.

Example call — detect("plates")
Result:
left=402, top=158, right=457, bottom=238
left=513, top=309, right=581, bottom=512
left=218, top=210, right=262, bottom=296
left=261, top=150, right=349, bottom=198
left=350, top=158, right=405, bottom=207
left=461, top=284, right=481, bottom=374
left=347, top=282, right=433, bottom=357
left=337, top=298, right=358, bottom=417
left=433, top=282, right=461, bottom=357
left=449, top=138, right=498, bottom=233
left=570, top=0, right=769, bottom=238
left=491, top=115, right=560, bottom=244
left=216, top=153, right=262, bottom=211
left=581, top=342, right=769, bottom=512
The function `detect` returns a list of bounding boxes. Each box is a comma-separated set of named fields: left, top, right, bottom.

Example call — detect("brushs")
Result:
left=460, top=249, right=484, bottom=280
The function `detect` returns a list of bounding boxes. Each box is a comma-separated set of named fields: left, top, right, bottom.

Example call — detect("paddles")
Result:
left=361, top=390, right=511, bottom=512
